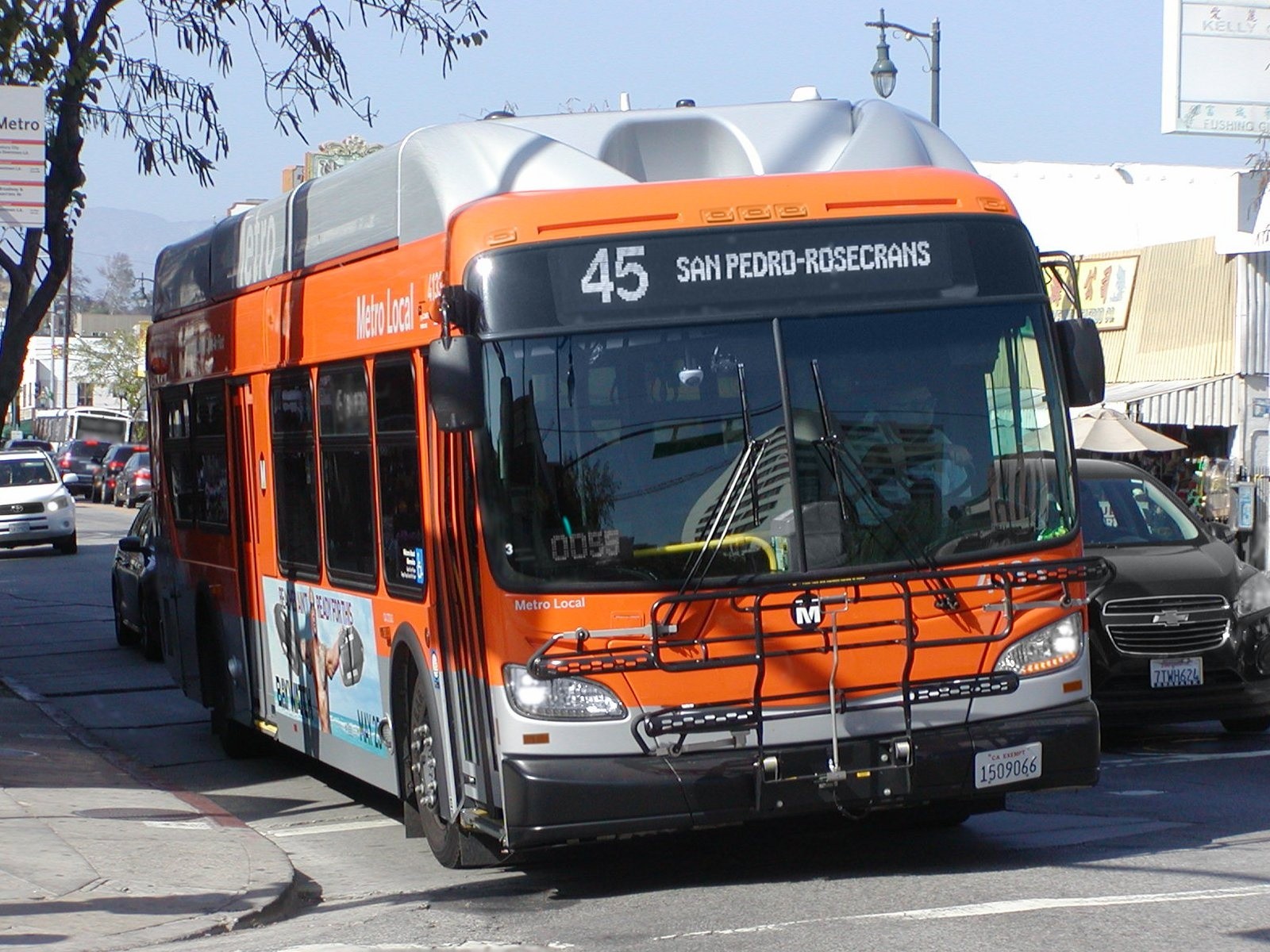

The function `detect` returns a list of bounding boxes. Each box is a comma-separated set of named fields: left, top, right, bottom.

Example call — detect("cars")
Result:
left=111, top=452, right=154, bottom=508
left=111, top=495, right=167, bottom=666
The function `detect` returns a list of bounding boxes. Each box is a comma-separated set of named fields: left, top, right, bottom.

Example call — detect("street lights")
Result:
left=864, top=8, right=941, bottom=130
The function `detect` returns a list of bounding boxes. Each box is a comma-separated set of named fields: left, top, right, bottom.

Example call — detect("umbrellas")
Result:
left=989, top=415, right=1032, bottom=457
left=1022, top=403, right=1188, bottom=453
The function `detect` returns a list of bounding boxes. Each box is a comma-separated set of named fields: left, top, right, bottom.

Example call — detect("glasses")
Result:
left=897, top=397, right=939, bottom=414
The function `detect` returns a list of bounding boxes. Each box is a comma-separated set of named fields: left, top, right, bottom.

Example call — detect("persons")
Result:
left=846, top=373, right=971, bottom=565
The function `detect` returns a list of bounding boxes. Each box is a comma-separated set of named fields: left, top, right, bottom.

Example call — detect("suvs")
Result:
left=0, top=445, right=80, bottom=555
left=991, top=457, right=1270, bottom=752
left=89, top=443, right=150, bottom=504
left=4, top=439, right=61, bottom=475
left=47, top=439, right=112, bottom=499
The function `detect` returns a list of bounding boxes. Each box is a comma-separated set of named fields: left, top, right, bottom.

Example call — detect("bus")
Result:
left=138, top=97, right=1107, bottom=879
left=33, top=406, right=136, bottom=443
